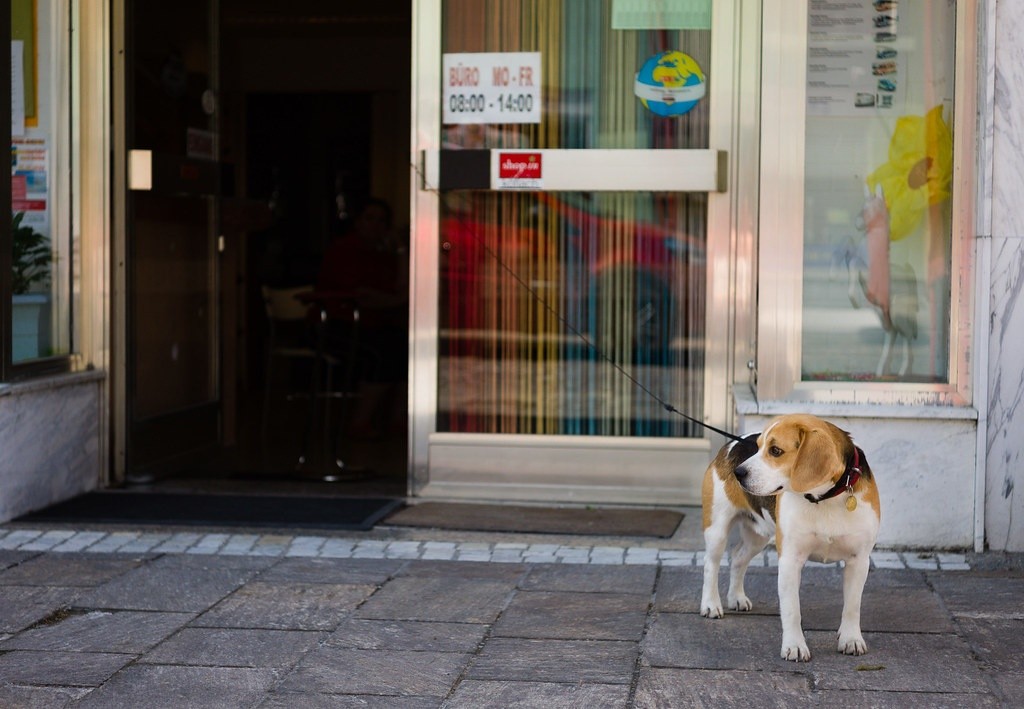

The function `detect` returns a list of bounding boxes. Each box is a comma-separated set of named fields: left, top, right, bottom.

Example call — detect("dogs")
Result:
left=700, top=413, right=882, bottom=663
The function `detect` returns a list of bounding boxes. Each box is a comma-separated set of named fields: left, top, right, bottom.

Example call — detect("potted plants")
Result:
left=8, top=209, right=50, bottom=363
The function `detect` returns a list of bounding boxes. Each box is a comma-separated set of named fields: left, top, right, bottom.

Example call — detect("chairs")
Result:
left=258, top=285, right=365, bottom=437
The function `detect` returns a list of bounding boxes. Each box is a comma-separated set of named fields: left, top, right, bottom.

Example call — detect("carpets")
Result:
left=385, top=500, right=685, bottom=541
left=0, top=488, right=406, bottom=532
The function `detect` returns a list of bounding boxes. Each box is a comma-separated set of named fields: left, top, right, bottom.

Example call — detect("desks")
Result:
left=295, top=290, right=410, bottom=476
